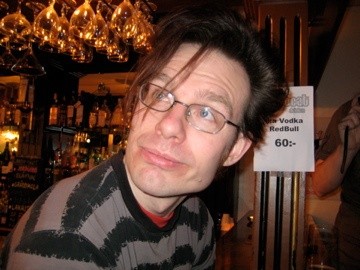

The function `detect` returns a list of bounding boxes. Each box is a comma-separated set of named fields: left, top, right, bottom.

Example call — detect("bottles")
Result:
left=0, top=84, right=136, bottom=231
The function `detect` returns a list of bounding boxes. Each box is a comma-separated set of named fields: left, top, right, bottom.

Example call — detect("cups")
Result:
left=303, top=222, right=340, bottom=270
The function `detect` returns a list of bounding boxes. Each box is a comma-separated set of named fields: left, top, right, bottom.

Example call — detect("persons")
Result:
left=4, top=2, right=291, bottom=270
left=311, top=87, right=360, bottom=270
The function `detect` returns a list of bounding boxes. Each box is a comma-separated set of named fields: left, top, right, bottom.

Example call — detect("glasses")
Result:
left=139, top=82, right=243, bottom=135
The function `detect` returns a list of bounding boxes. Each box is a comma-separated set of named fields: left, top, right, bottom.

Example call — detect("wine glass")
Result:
left=0, top=1, right=161, bottom=76
